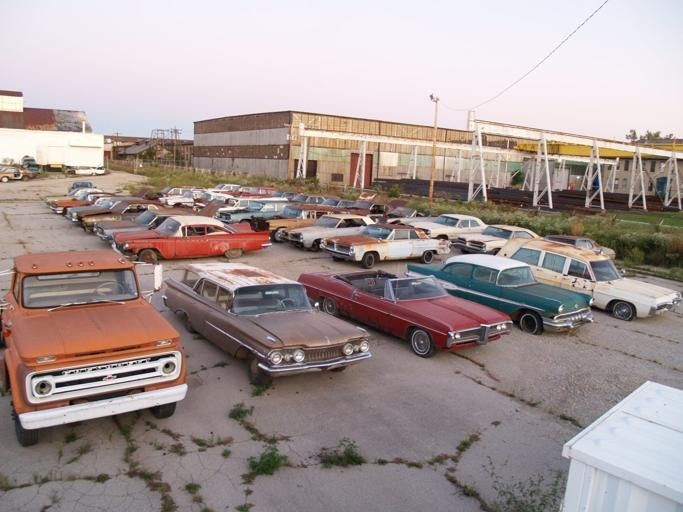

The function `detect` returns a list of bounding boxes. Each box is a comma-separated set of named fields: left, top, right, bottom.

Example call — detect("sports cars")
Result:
left=297, top=265, right=514, bottom=363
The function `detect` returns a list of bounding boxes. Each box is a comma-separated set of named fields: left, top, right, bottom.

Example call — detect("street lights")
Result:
left=429, top=86, right=441, bottom=200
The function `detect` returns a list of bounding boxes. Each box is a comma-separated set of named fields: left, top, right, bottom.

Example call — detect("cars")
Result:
left=44, top=182, right=427, bottom=234
left=408, top=213, right=490, bottom=240
left=161, top=257, right=374, bottom=393
left=278, top=213, right=379, bottom=253
left=407, top=251, right=596, bottom=339
left=452, top=224, right=547, bottom=255
left=319, top=223, right=452, bottom=269
left=108, top=215, right=272, bottom=264
left=496, top=234, right=680, bottom=328
left=0, top=156, right=104, bottom=183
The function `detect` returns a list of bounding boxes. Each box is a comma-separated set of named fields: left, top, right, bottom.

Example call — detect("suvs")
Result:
left=2, top=245, right=189, bottom=450
